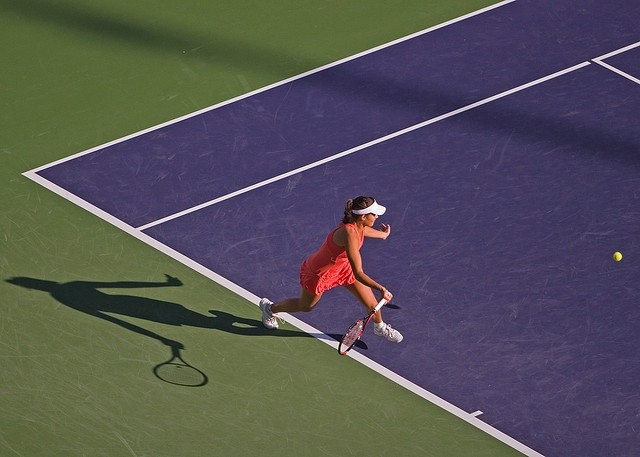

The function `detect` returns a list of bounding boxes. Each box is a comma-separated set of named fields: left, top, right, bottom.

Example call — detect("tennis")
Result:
left=613, top=252, right=622, bottom=262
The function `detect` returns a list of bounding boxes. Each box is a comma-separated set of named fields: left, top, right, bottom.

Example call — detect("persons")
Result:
left=259, top=196, right=403, bottom=344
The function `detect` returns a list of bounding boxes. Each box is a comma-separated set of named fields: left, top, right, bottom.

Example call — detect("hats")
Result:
left=351, top=196, right=387, bottom=215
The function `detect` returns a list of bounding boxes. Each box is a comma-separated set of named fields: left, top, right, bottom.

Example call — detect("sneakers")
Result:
left=259, top=297, right=285, bottom=330
left=373, top=322, right=404, bottom=343
